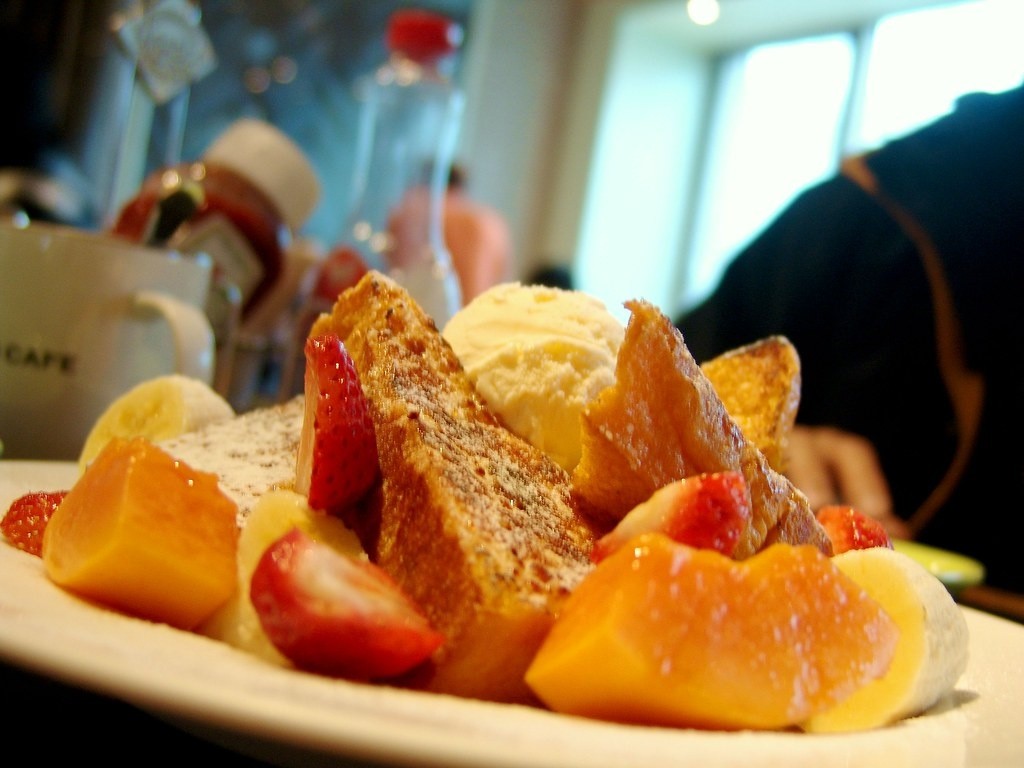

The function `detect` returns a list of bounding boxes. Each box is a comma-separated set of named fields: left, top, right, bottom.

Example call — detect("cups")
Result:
left=1, top=217, right=216, bottom=458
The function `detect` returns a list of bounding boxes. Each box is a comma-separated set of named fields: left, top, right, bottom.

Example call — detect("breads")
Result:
left=316, top=270, right=626, bottom=709
left=572, top=296, right=833, bottom=553
left=693, top=334, right=803, bottom=472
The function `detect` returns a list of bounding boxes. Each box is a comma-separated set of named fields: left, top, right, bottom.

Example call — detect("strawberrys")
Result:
left=816, top=502, right=893, bottom=555
left=592, top=469, right=756, bottom=560
left=245, top=530, right=447, bottom=678
left=294, top=330, right=380, bottom=511
left=0, top=490, right=70, bottom=556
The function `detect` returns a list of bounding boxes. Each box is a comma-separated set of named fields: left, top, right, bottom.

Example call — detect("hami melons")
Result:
left=520, top=532, right=904, bottom=729
left=41, top=438, right=243, bottom=629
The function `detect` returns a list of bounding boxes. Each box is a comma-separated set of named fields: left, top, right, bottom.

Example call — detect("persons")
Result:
left=387, top=160, right=507, bottom=309
left=673, top=86, right=1024, bottom=627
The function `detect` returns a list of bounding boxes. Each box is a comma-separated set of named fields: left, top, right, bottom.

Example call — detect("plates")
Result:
left=0, top=457, right=1024, bottom=766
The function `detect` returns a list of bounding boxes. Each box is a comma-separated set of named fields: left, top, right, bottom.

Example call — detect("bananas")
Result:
left=76, top=374, right=237, bottom=454
left=796, top=548, right=968, bottom=732
left=206, top=490, right=386, bottom=664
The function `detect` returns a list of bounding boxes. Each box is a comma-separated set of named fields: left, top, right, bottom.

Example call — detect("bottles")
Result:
left=281, top=14, right=461, bottom=417
left=108, top=116, right=320, bottom=397
